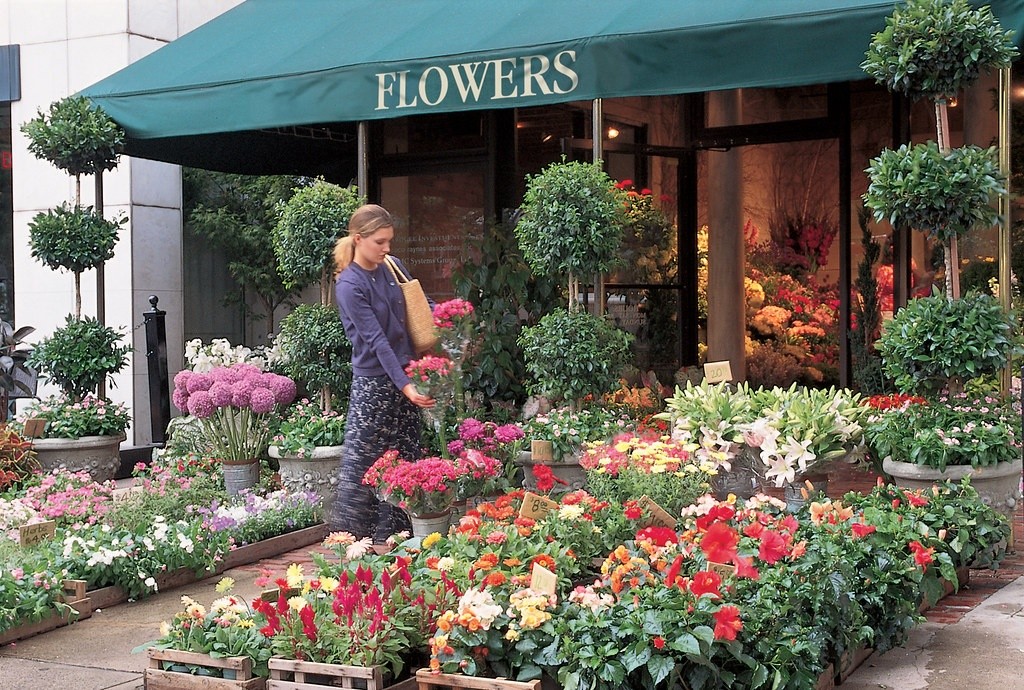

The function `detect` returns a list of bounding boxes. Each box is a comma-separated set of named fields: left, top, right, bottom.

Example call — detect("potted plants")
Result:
left=514, top=153, right=652, bottom=492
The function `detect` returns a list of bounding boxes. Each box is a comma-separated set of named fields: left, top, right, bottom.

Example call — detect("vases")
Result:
left=410, top=517, right=450, bottom=541
left=268, top=444, right=348, bottom=504
left=223, top=463, right=261, bottom=495
left=878, top=454, right=1023, bottom=567
left=11, top=436, right=127, bottom=485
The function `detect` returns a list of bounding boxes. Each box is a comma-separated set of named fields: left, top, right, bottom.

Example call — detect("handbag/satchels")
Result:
left=383, top=254, right=439, bottom=353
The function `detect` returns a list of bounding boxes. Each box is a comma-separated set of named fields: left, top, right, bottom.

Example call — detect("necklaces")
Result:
left=352, top=260, right=378, bottom=282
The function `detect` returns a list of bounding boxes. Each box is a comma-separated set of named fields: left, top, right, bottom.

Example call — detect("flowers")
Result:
left=172, top=363, right=297, bottom=462
left=360, top=451, right=467, bottom=516
left=447, top=416, right=525, bottom=456
left=531, top=378, right=1024, bottom=488
left=145, top=490, right=1011, bottom=690
left=614, top=181, right=672, bottom=245
left=284, top=401, right=342, bottom=441
left=0, top=467, right=328, bottom=643
left=612, top=178, right=933, bottom=390
left=28, top=393, right=127, bottom=434
left=403, top=297, right=475, bottom=457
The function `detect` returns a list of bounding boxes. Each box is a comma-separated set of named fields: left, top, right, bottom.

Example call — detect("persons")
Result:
left=333, top=205, right=454, bottom=554
